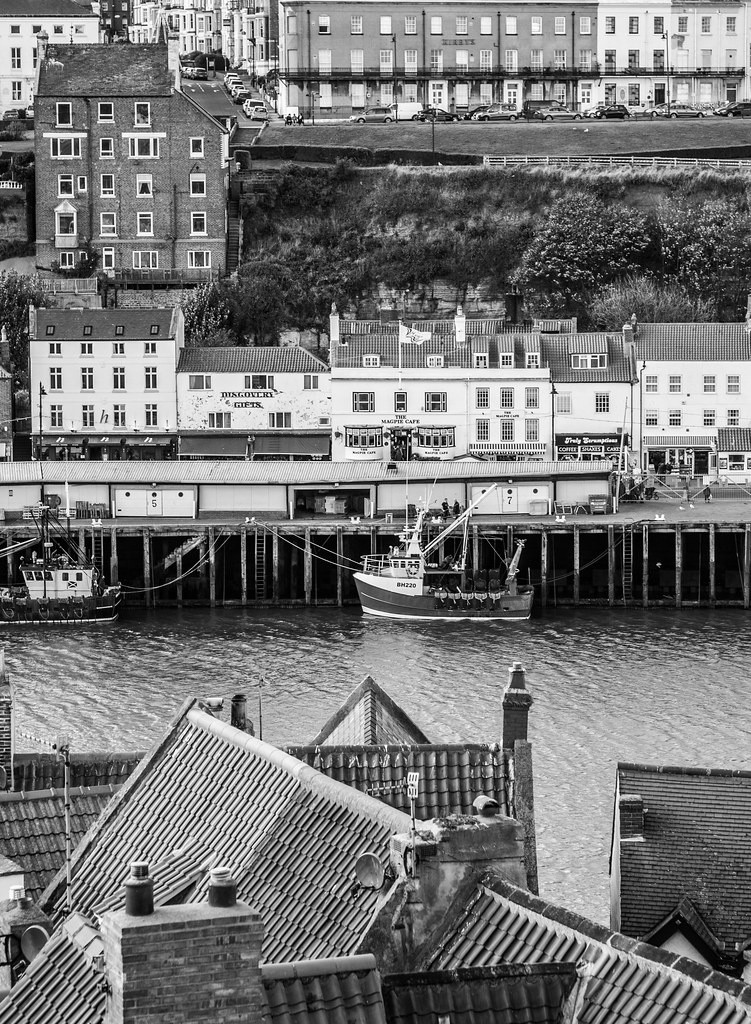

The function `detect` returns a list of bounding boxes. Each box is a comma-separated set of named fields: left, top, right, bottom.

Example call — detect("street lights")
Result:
left=639, top=360, right=646, bottom=475
left=549, top=381, right=559, bottom=461
left=391, top=32, right=399, bottom=123
left=39, top=381, right=48, bottom=461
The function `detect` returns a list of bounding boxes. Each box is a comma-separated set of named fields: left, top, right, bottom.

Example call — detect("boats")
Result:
left=0, top=506, right=122, bottom=624
left=353, top=436, right=535, bottom=620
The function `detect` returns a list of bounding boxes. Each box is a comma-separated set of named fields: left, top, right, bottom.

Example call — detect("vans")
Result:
left=524, top=100, right=567, bottom=119
left=386, top=102, right=423, bottom=121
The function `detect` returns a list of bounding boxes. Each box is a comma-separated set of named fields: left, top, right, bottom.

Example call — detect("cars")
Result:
left=645, top=102, right=707, bottom=119
left=223, top=72, right=269, bottom=121
left=350, top=107, right=394, bottom=124
left=584, top=104, right=632, bottom=119
left=420, top=108, right=458, bottom=122
left=181, top=67, right=208, bottom=81
left=540, top=107, right=583, bottom=120
left=715, top=102, right=751, bottom=117
left=464, top=104, right=518, bottom=121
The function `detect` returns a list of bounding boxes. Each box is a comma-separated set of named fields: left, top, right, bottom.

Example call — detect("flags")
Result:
left=400, top=325, right=431, bottom=347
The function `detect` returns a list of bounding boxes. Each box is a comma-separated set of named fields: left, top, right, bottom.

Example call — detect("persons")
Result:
left=703, top=485, right=712, bottom=503
left=453, top=499, right=460, bottom=519
left=441, top=498, right=450, bottom=523
